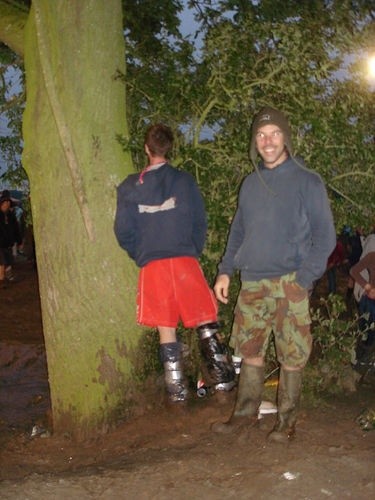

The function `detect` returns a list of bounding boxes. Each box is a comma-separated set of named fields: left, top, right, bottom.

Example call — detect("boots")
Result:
left=266, top=368, right=302, bottom=445
left=197, top=323, right=236, bottom=389
left=160, top=340, right=187, bottom=406
left=210, top=362, right=265, bottom=435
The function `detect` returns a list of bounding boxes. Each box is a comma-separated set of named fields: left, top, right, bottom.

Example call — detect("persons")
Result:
left=343, top=226, right=366, bottom=301
left=326, top=241, right=342, bottom=294
left=114, top=125, right=238, bottom=403
left=349, top=232, right=375, bottom=348
left=212, top=107, right=336, bottom=443
left=0, top=190, right=19, bottom=289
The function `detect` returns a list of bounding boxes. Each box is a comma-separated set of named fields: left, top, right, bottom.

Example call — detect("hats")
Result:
left=249, top=105, right=295, bottom=164
